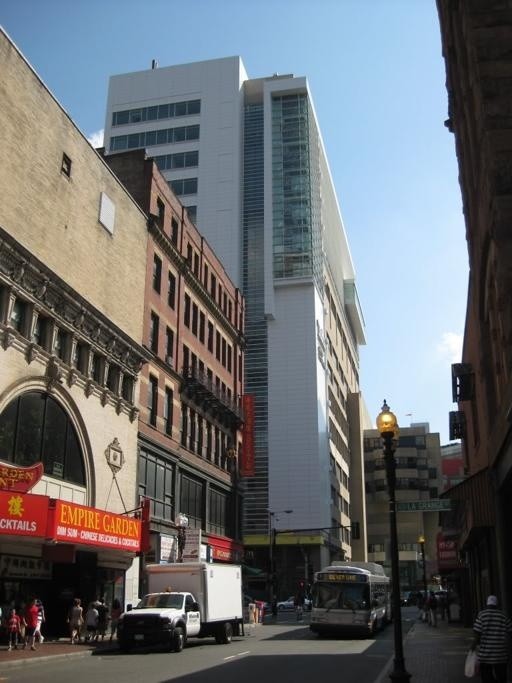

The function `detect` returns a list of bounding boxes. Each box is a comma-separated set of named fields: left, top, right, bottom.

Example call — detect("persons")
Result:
left=248, top=595, right=278, bottom=628
left=294, top=587, right=307, bottom=623
left=1, top=597, right=134, bottom=651
left=402, top=587, right=462, bottom=627
left=467, top=594, right=511, bottom=683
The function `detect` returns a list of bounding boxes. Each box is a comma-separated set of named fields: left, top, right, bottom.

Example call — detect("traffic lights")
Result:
left=298, top=579, right=305, bottom=590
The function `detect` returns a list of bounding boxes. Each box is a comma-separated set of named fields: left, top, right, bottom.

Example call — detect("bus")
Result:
left=310, top=561, right=393, bottom=638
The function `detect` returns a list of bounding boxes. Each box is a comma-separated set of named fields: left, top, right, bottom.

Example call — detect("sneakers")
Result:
left=6, top=637, right=46, bottom=653
left=71, top=635, right=114, bottom=644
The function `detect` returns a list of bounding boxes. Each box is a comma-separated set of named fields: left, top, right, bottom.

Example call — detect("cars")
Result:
left=276, top=596, right=313, bottom=609
left=400, top=591, right=416, bottom=606
left=435, top=590, right=447, bottom=601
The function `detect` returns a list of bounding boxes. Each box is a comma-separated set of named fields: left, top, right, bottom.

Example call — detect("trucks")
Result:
left=118, top=563, right=244, bottom=652
left=244, top=573, right=280, bottom=614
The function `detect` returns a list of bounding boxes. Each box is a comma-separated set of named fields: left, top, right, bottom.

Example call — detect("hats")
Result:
left=486, top=595, right=498, bottom=606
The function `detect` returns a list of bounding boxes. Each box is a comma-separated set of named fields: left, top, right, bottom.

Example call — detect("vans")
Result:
left=417, top=591, right=435, bottom=608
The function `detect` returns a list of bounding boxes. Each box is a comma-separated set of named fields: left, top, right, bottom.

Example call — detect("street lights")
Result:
left=418, top=535, right=427, bottom=596
left=265, top=509, right=293, bottom=577
left=375, top=399, right=411, bottom=683
left=175, top=512, right=189, bottom=563
